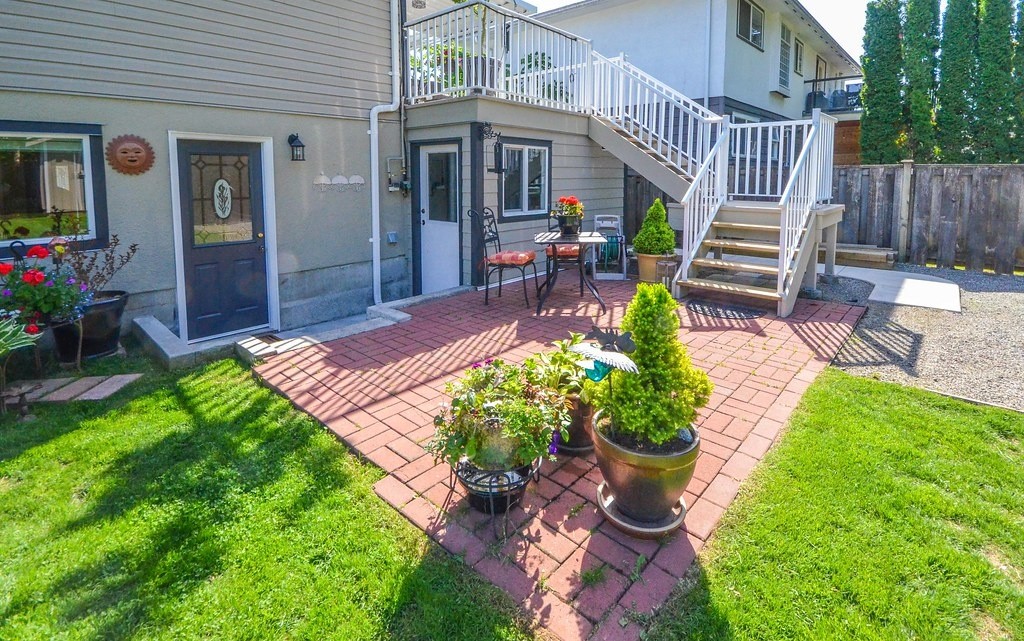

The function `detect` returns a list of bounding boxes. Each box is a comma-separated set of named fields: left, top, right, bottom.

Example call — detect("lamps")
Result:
left=288, top=133, right=306, bottom=161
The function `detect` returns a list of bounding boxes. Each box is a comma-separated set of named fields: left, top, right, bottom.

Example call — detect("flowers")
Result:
left=0, top=245, right=93, bottom=333
left=550, top=195, right=585, bottom=220
left=422, top=356, right=562, bottom=470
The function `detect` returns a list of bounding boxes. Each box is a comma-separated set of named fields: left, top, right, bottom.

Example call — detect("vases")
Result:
left=557, top=215, right=581, bottom=237
left=455, top=458, right=534, bottom=515
left=5, top=329, right=44, bottom=380
left=47, top=312, right=88, bottom=370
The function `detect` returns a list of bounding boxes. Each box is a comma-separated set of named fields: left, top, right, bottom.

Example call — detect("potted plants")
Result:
left=36, top=205, right=141, bottom=362
left=521, top=330, right=599, bottom=457
left=631, top=198, right=677, bottom=282
left=582, top=284, right=717, bottom=540
left=453, top=0, right=503, bottom=93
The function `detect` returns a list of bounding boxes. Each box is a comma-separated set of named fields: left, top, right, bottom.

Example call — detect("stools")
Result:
left=656, top=260, right=677, bottom=289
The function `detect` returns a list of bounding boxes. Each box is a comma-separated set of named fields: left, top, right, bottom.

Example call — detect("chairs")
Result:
left=467, top=206, right=542, bottom=309
left=545, top=203, right=587, bottom=297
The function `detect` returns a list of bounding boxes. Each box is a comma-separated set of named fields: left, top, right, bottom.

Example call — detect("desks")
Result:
left=533, top=231, right=609, bottom=315
